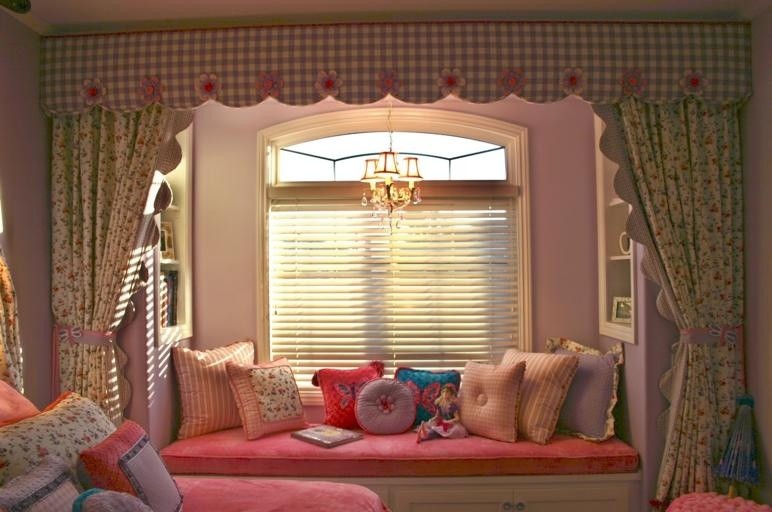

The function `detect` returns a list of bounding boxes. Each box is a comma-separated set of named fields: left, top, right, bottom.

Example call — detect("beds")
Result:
left=159, top=423, right=639, bottom=512
left=0, top=377, right=392, bottom=512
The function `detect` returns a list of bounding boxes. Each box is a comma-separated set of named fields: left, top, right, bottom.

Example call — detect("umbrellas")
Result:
left=714, top=395, right=760, bottom=497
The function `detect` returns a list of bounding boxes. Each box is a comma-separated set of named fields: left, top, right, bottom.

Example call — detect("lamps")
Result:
left=360, top=102, right=422, bottom=234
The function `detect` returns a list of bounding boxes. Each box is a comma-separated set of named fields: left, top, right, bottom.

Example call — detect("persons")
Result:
left=414, top=382, right=470, bottom=444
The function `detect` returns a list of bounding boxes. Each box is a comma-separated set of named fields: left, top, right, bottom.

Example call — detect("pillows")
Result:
left=459, top=336, right=624, bottom=443
left=312, top=361, right=385, bottom=429
left=171, top=339, right=254, bottom=441
left=354, top=377, right=416, bottom=435
left=0, top=392, right=185, bottom=512
left=223, top=355, right=308, bottom=441
left=393, top=366, right=461, bottom=428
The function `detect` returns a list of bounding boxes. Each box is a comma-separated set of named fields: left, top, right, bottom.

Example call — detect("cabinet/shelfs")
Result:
left=593, top=115, right=636, bottom=344
left=389, top=476, right=629, bottom=512
left=155, top=122, right=193, bottom=348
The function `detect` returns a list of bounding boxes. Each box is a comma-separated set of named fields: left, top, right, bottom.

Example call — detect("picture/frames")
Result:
left=611, top=296, right=632, bottom=324
left=160, top=221, right=175, bottom=258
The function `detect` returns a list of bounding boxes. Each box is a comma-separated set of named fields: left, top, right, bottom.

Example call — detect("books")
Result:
left=290, top=424, right=363, bottom=448
